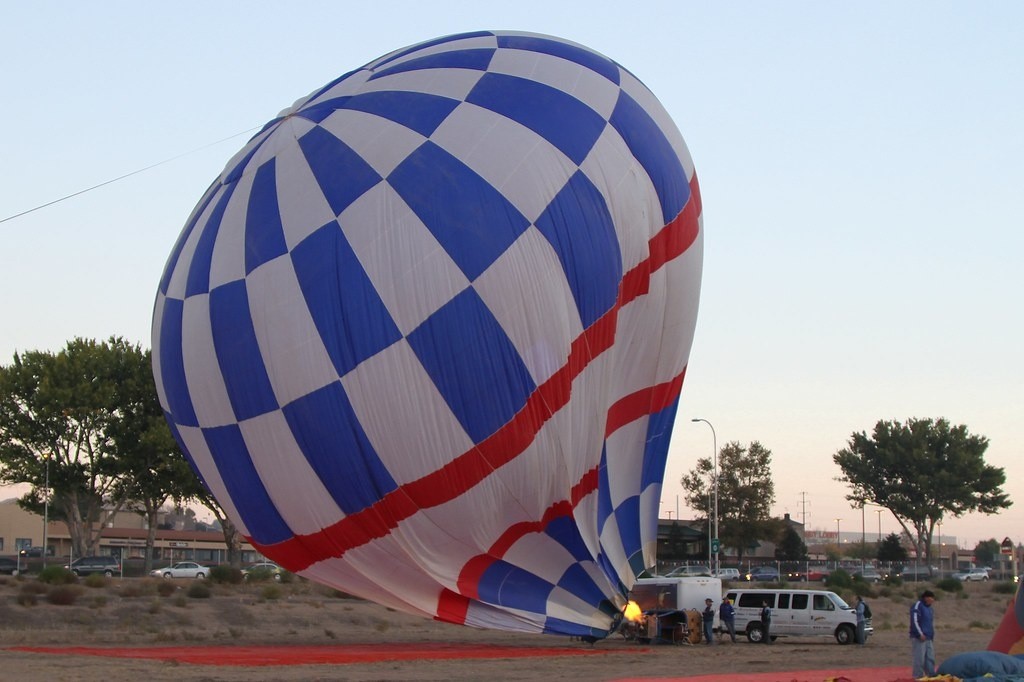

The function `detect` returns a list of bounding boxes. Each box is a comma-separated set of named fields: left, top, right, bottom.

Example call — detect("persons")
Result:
left=911, top=591, right=939, bottom=678
left=761, top=600, right=772, bottom=645
left=720, top=597, right=739, bottom=644
left=852, top=595, right=868, bottom=645
left=702, top=598, right=716, bottom=646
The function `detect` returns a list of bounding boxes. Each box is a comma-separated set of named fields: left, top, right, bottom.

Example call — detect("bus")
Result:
left=720, top=589, right=874, bottom=645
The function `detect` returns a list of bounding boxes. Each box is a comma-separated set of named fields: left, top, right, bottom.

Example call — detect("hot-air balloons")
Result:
left=150, top=28, right=705, bottom=644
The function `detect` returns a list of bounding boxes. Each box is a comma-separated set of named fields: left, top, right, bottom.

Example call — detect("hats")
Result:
left=921, top=591, right=934, bottom=597
left=704, top=599, right=713, bottom=602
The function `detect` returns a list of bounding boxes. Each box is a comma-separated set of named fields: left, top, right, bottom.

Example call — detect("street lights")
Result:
left=936, top=520, right=944, bottom=580
left=834, top=518, right=843, bottom=568
left=692, top=418, right=718, bottom=576
left=875, top=509, right=885, bottom=541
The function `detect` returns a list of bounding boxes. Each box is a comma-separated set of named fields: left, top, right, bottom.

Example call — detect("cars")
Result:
left=20, top=546, right=53, bottom=557
left=0, top=558, right=31, bottom=576
left=666, top=565, right=711, bottom=576
left=149, top=561, right=211, bottom=579
left=740, top=566, right=779, bottom=581
left=787, top=565, right=831, bottom=582
left=63, top=556, right=120, bottom=577
left=127, top=555, right=145, bottom=560
left=952, top=568, right=989, bottom=583
left=241, top=563, right=282, bottom=584
left=849, top=570, right=881, bottom=582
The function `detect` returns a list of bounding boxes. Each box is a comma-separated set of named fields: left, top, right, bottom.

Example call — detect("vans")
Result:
left=712, top=568, right=740, bottom=582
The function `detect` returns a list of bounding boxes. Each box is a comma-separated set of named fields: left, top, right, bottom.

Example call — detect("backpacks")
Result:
left=860, top=602, right=872, bottom=618
